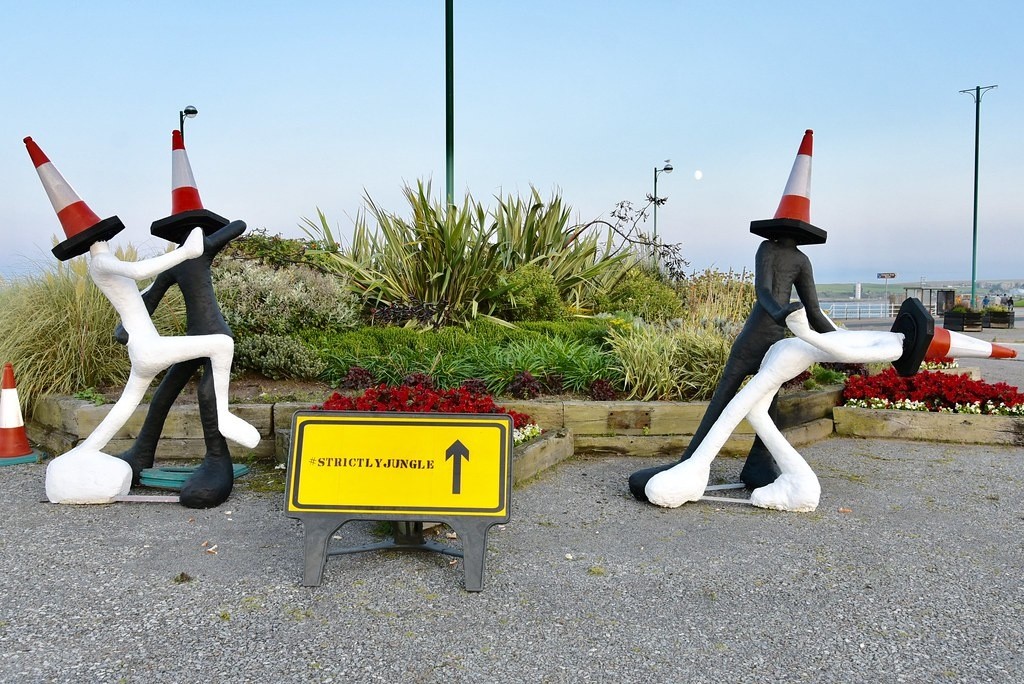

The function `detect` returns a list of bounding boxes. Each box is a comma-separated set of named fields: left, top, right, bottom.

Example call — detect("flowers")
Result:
left=309, top=381, right=544, bottom=446
left=841, top=357, right=1024, bottom=416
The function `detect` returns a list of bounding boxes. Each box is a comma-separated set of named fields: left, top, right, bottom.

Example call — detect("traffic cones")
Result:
left=749, top=126, right=828, bottom=246
left=146, top=124, right=231, bottom=246
left=890, top=297, right=1020, bottom=377
left=21, top=134, right=125, bottom=261
left=0, top=361, right=42, bottom=465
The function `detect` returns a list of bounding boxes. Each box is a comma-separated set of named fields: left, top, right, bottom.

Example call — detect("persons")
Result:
left=982, top=296, right=990, bottom=307
left=995, top=294, right=1013, bottom=311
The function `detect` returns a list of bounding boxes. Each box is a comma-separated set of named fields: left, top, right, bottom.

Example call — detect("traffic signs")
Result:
left=282, top=410, right=516, bottom=524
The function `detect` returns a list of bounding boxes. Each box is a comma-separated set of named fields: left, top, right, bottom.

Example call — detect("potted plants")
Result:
left=982, top=304, right=1015, bottom=328
left=943, top=304, right=984, bottom=332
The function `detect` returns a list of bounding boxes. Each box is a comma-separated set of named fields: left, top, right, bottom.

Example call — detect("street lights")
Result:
left=651, top=164, right=674, bottom=263
left=959, top=83, right=996, bottom=312
